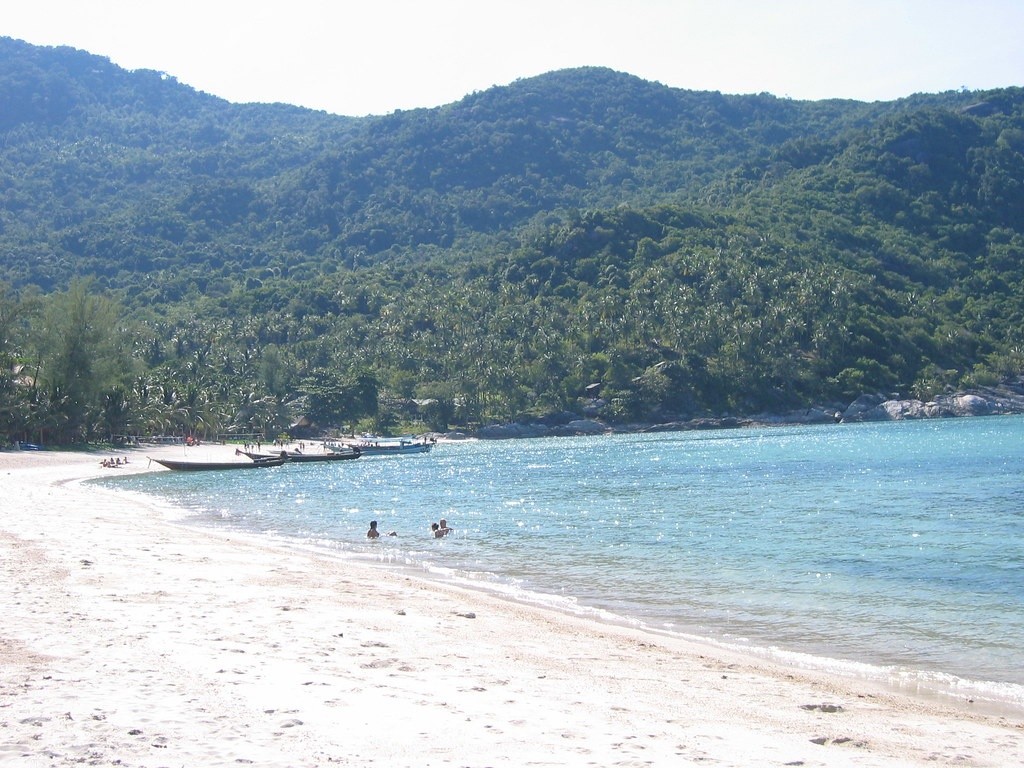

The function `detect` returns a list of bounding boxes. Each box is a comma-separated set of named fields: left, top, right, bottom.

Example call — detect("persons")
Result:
left=103, top=456, right=127, bottom=468
left=244, top=442, right=260, bottom=453
left=432, top=519, right=453, bottom=539
left=273, top=435, right=304, bottom=450
left=367, top=520, right=379, bottom=539
left=310, top=434, right=370, bottom=448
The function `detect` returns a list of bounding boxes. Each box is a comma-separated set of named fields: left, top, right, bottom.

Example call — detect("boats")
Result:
left=320, top=438, right=435, bottom=455
left=145, top=451, right=292, bottom=470
left=235, top=446, right=365, bottom=462
